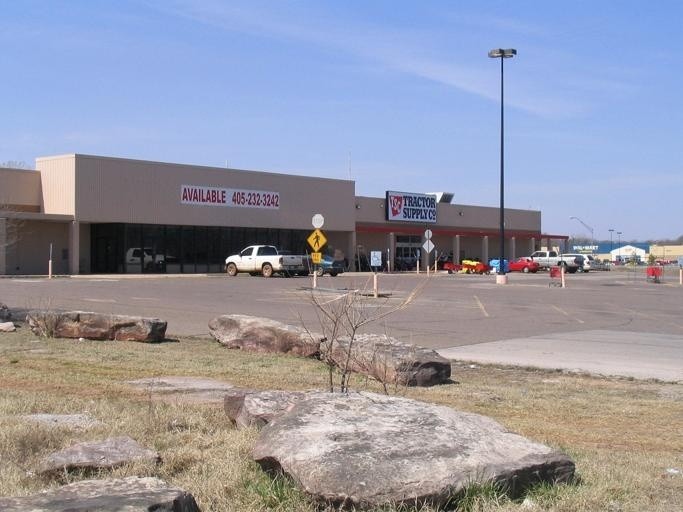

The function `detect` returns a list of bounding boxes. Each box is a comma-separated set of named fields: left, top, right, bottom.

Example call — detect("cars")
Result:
left=443, top=251, right=678, bottom=276
left=294, top=254, right=344, bottom=276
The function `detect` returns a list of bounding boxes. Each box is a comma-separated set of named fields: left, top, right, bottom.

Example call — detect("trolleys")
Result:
left=548, top=264, right=562, bottom=288
left=646, top=260, right=662, bottom=284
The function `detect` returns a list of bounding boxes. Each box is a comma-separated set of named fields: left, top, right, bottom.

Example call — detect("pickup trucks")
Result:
left=224, top=245, right=306, bottom=278
left=126, top=246, right=178, bottom=273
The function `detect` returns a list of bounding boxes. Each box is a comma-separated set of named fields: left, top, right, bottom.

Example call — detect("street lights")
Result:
left=617, top=231, right=622, bottom=255
left=608, top=229, right=614, bottom=251
left=569, top=216, right=594, bottom=254
left=488, top=49, right=516, bottom=284
